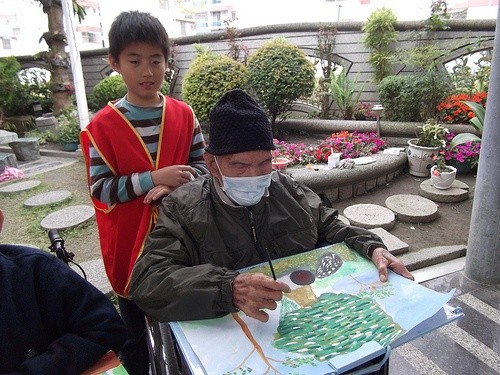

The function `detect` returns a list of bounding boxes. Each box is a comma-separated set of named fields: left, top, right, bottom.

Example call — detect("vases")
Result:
left=406, top=138, right=442, bottom=179
left=450, top=157, right=476, bottom=176
left=431, top=165, right=457, bottom=190
left=271, top=158, right=290, bottom=175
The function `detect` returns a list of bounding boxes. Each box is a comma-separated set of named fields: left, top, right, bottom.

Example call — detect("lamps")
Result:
left=31, top=101, right=43, bottom=117
left=371, top=104, right=385, bottom=115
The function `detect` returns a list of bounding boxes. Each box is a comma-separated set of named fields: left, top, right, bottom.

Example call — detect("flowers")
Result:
left=416, top=90, right=488, bottom=177
left=271, top=101, right=385, bottom=172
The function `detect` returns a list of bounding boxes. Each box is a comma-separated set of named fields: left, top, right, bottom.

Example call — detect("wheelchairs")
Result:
left=143, top=172, right=390, bottom=375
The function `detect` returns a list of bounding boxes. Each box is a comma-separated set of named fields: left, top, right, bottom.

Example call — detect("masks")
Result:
left=213, top=156, right=273, bottom=206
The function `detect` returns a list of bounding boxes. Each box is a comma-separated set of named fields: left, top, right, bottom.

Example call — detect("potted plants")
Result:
left=59, top=132, right=80, bottom=152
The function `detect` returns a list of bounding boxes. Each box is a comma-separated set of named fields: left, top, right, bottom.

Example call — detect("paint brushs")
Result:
left=266, top=248, right=284, bottom=306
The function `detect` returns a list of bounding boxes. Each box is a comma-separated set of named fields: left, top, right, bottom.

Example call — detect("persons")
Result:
left=78, top=11, right=211, bottom=375
left=127, top=90, right=414, bottom=375
left=0, top=207, right=125, bottom=375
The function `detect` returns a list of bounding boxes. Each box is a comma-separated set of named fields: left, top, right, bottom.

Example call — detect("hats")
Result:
left=204, top=90, right=275, bottom=155
